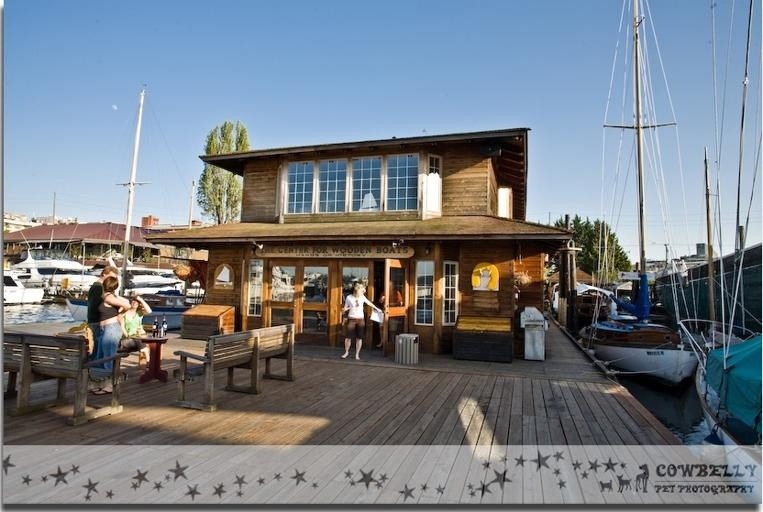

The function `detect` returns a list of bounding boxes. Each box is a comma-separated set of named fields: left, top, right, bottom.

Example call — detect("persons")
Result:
left=86, top=266, right=121, bottom=395
left=340, top=282, right=384, bottom=362
left=91, top=275, right=131, bottom=395
left=376, top=281, right=404, bottom=349
left=119, top=294, right=151, bottom=369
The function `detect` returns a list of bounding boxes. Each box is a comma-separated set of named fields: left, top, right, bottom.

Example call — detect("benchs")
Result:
left=3, top=331, right=128, bottom=427
left=172, top=323, right=298, bottom=412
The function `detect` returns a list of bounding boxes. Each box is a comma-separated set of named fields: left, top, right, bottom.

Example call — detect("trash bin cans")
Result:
left=520, top=306, right=548, bottom=361
left=394, top=333, right=419, bottom=366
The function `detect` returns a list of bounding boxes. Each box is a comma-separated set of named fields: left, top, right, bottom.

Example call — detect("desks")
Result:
left=130, top=332, right=168, bottom=383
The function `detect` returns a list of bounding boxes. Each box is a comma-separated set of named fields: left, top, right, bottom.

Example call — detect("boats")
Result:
left=4, top=246, right=205, bottom=332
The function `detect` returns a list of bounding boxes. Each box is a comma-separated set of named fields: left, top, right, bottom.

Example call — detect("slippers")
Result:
left=88, top=387, right=114, bottom=395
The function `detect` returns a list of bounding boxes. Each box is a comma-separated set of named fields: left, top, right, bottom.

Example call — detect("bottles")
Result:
left=162, top=313, right=168, bottom=336
left=152, top=314, right=159, bottom=338
